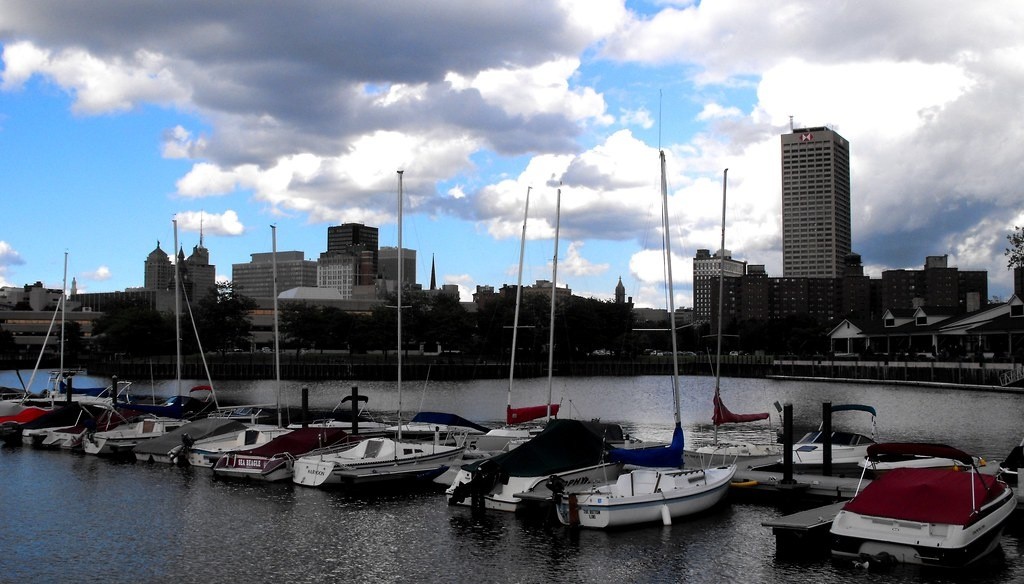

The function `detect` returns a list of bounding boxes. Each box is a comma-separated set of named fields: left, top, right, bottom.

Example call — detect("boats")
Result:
left=776, top=428, right=881, bottom=471
left=826, top=440, right=1016, bottom=573
left=997, top=439, right=1024, bottom=509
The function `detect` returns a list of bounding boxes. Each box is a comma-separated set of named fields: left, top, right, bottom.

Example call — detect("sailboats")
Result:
left=666, top=169, right=783, bottom=463
left=0, top=168, right=626, bottom=515
left=513, top=148, right=737, bottom=529
